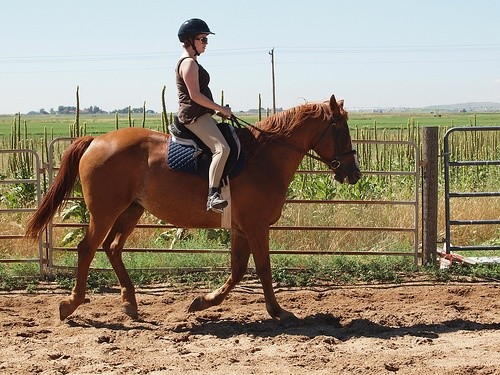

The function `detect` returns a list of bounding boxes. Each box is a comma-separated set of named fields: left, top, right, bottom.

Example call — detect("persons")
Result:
left=174, top=18, right=233, bottom=212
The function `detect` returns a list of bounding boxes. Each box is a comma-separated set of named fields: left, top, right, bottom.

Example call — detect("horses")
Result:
left=21, top=94, right=363, bottom=328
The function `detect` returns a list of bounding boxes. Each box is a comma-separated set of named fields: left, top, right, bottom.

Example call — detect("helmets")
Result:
left=178, top=18, right=215, bottom=36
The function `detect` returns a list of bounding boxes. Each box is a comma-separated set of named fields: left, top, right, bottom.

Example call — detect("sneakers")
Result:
left=207, top=196, right=228, bottom=212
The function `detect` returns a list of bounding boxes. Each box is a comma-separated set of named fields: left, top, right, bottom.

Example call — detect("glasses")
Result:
left=193, top=38, right=207, bottom=44
